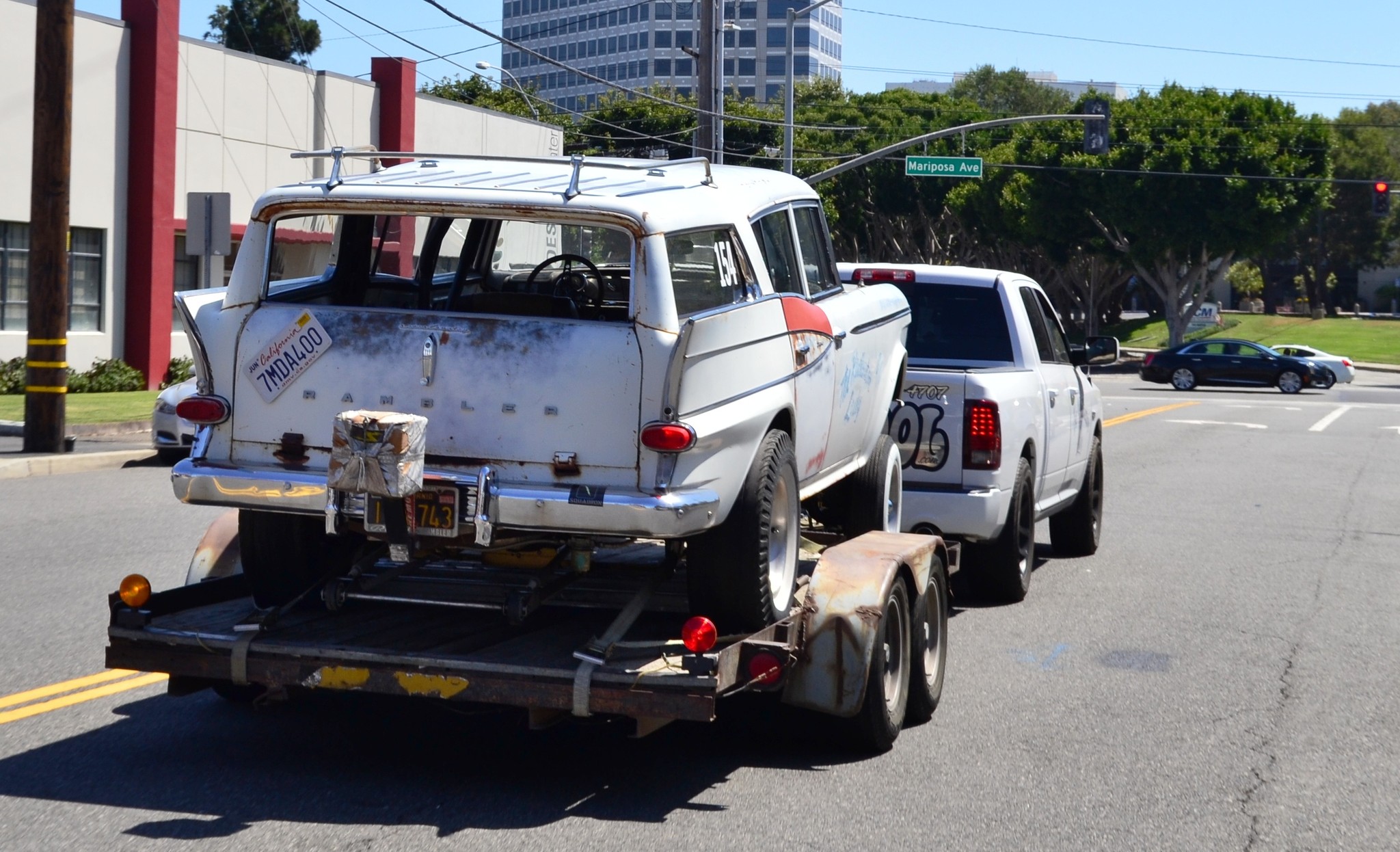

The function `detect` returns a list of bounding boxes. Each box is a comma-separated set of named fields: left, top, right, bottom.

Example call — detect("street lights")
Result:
left=476, top=60, right=541, bottom=121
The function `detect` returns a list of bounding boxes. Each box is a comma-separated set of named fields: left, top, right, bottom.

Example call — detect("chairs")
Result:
left=332, top=281, right=432, bottom=311
left=475, top=292, right=581, bottom=320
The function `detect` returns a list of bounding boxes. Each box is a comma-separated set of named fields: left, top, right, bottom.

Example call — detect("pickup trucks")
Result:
left=792, top=260, right=1106, bottom=608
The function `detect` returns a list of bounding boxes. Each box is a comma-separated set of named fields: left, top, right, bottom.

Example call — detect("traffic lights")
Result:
left=1370, top=182, right=1391, bottom=217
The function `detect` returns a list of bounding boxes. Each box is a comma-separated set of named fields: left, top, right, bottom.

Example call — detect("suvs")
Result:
left=170, top=152, right=918, bottom=637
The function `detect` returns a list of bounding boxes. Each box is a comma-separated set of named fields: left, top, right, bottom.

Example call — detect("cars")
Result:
left=1267, top=344, right=1355, bottom=390
left=150, top=365, right=197, bottom=463
left=1136, top=337, right=1332, bottom=394
